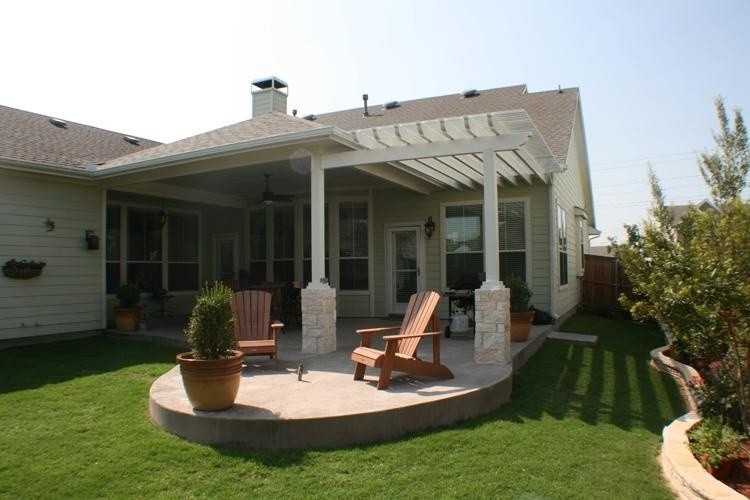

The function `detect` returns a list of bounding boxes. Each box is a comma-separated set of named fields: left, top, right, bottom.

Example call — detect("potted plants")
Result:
left=176, top=279, right=246, bottom=413
left=111, top=282, right=144, bottom=332
left=502, top=271, right=534, bottom=342
left=2, top=259, right=46, bottom=279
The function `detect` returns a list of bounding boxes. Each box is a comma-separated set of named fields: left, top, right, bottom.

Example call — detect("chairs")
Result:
left=350, top=290, right=455, bottom=391
left=228, top=289, right=285, bottom=361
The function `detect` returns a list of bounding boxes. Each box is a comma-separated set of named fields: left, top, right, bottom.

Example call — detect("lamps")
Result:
left=424, top=216, right=435, bottom=239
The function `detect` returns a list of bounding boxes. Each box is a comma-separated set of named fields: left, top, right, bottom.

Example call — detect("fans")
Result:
left=236, top=173, right=294, bottom=206
